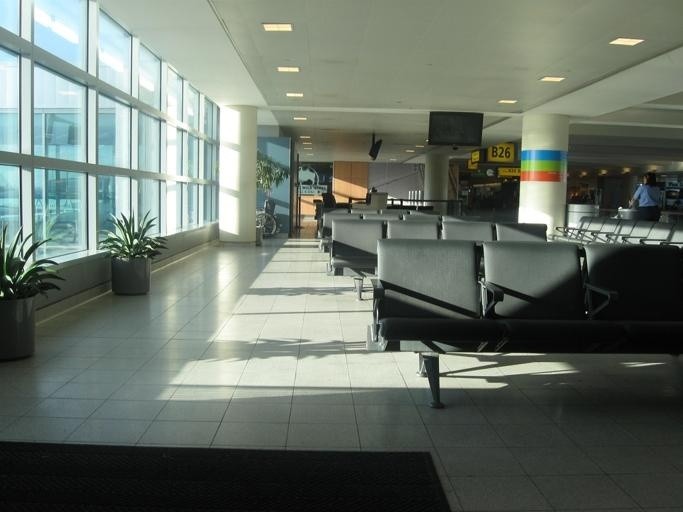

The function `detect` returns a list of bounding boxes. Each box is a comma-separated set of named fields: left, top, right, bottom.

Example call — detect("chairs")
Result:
left=326, top=218, right=549, bottom=302
left=316, top=203, right=484, bottom=255
left=361, top=235, right=681, bottom=408
left=547, top=215, right=683, bottom=249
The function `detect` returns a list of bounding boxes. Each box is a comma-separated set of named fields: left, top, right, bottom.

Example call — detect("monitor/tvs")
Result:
left=428, top=111, right=484, bottom=146
left=369, top=139, right=384, bottom=160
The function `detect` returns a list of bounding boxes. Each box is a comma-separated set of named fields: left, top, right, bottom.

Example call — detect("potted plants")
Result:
left=0, top=223, right=67, bottom=361
left=97, top=209, right=169, bottom=296
left=256, top=159, right=289, bottom=238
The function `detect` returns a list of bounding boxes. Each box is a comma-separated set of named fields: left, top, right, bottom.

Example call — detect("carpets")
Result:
left=0, top=441, right=452, bottom=512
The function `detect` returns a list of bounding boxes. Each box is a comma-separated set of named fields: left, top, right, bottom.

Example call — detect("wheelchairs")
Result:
left=256, top=199, right=277, bottom=236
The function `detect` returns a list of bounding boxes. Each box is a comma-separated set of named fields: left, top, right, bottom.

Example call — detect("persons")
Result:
left=628, top=172, right=661, bottom=222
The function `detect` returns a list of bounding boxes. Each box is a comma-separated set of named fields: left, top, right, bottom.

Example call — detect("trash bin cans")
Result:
left=256, top=225, right=264, bottom=246
